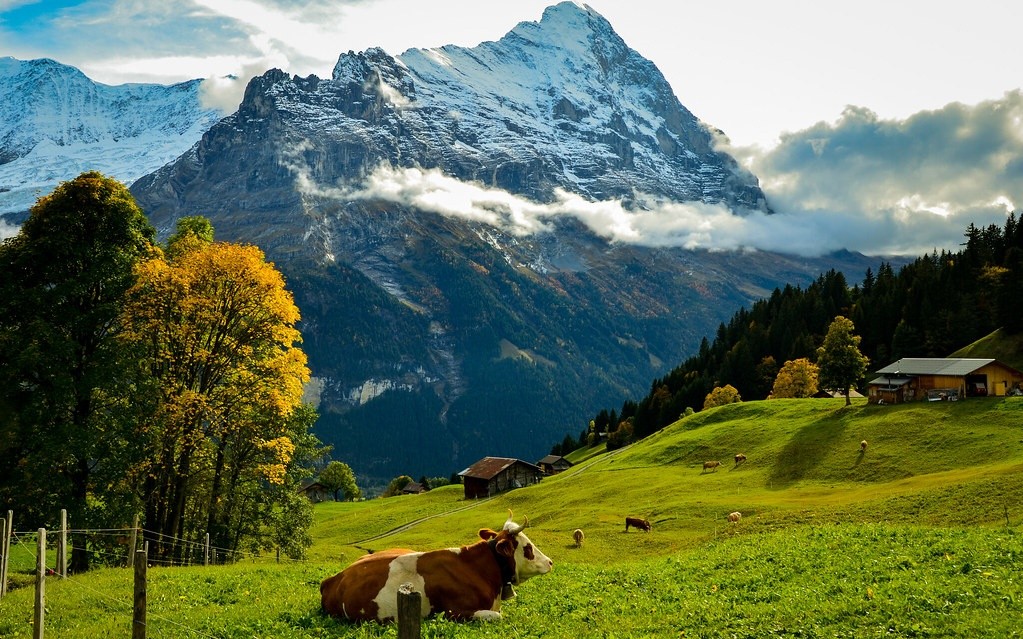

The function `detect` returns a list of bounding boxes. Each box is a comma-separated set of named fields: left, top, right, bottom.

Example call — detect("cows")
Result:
left=703, top=461, right=721, bottom=472
left=861, top=440, right=868, bottom=451
left=573, top=528, right=585, bottom=547
left=625, top=516, right=651, bottom=533
left=727, top=512, right=741, bottom=524
left=319, top=508, right=554, bottom=623
left=734, top=453, right=748, bottom=466
